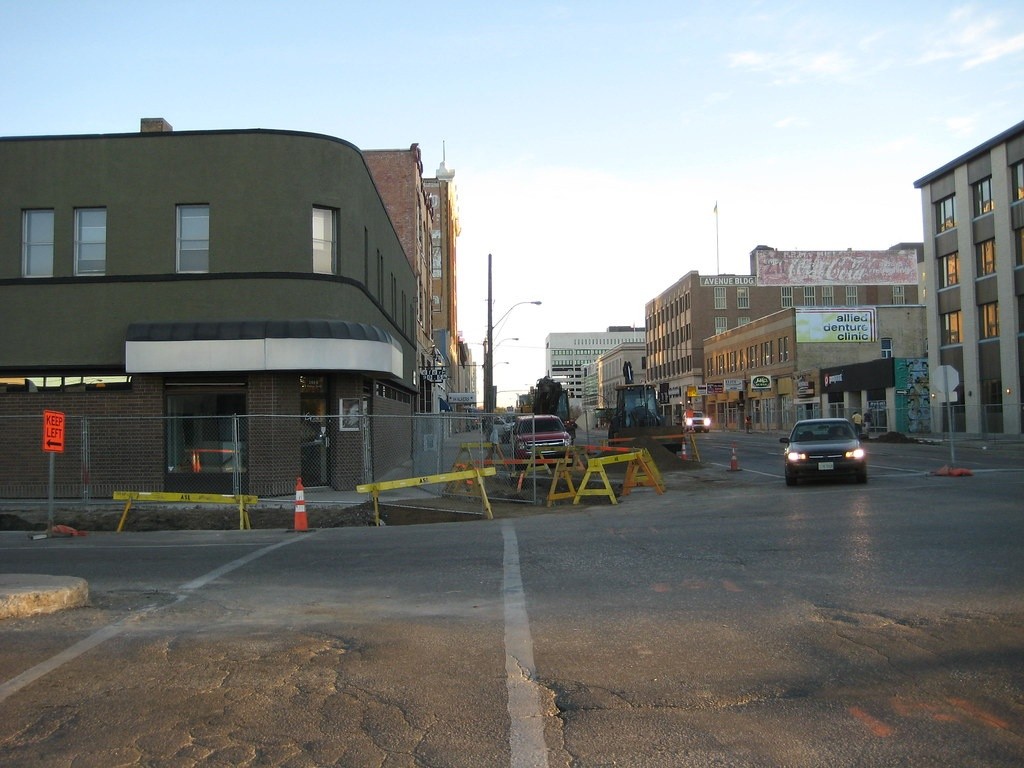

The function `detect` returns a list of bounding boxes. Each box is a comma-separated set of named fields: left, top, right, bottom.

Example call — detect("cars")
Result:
left=493, top=417, right=514, bottom=435
left=780, top=417, right=868, bottom=485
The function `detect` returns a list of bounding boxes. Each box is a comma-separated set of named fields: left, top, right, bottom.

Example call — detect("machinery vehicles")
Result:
left=608, top=360, right=686, bottom=454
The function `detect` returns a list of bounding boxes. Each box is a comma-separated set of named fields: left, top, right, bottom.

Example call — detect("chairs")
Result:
left=800, top=431, right=815, bottom=440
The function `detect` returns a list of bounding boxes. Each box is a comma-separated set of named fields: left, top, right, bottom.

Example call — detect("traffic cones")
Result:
left=283, top=477, right=316, bottom=533
left=726, top=443, right=742, bottom=472
left=679, top=439, right=689, bottom=460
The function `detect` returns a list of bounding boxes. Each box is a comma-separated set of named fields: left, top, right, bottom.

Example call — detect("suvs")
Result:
left=681, top=411, right=709, bottom=432
left=513, top=413, right=574, bottom=469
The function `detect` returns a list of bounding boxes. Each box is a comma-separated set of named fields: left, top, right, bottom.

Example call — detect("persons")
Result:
left=864, top=409, right=872, bottom=438
left=852, top=410, right=862, bottom=436
left=566, top=417, right=577, bottom=446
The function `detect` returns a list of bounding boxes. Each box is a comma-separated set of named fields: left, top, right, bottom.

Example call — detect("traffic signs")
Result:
left=43, top=409, right=64, bottom=454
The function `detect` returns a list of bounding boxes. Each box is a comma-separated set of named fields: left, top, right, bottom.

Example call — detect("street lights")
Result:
left=487, top=300, right=541, bottom=427
